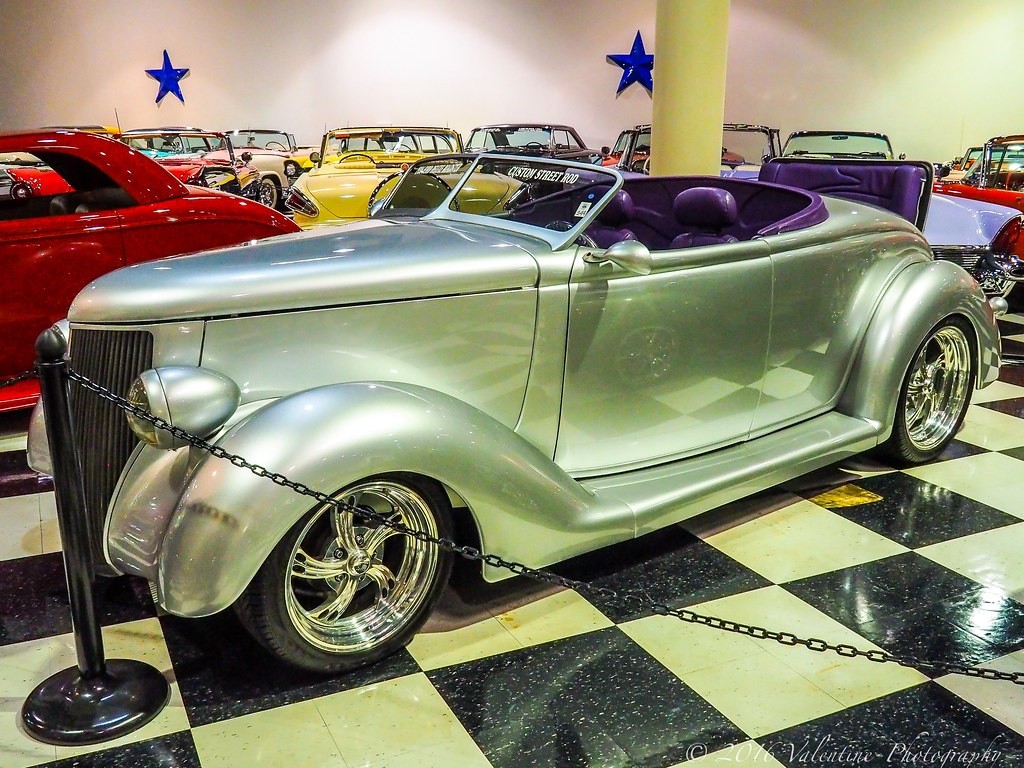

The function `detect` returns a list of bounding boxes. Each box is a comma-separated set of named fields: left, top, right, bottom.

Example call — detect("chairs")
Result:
left=581, top=185, right=638, bottom=248
left=667, top=187, right=738, bottom=248
left=50, top=196, right=73, bottom=216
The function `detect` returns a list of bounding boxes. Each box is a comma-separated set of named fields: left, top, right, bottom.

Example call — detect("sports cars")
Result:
left=38, top=124, right=1024, bottom=300
left=0, top=130, right=307, bottom=409
left=25, top=155, right=1005, bottom=677
